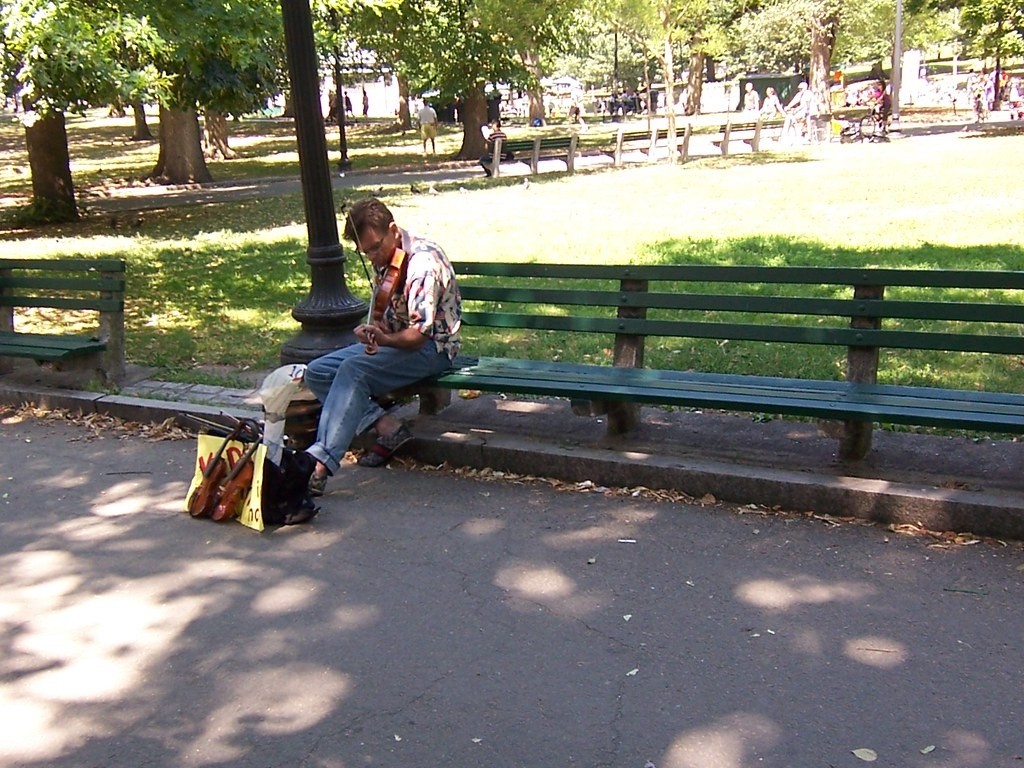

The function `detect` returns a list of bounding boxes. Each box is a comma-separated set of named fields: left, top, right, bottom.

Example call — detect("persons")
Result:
left=967, top=69, right=1023, bottom=123
left=412, top=99, right=438, bottom=155
left=325, top=89, right=368, bottom=122
left=305, top=198, right=462, bottom=494
left=610, top=90, right=642, bottom=116
left=742, top=79, right=891, bottom=141
left=479, top=119, right=507, bottom=178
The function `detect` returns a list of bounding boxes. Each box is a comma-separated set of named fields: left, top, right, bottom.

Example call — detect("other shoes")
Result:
left=308, top=474, right=328, bottom=494
left=358, top=424, right=415, bottom=467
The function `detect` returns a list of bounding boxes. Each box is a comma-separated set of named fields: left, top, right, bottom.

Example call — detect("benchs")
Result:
left=710, top=118, right=793, bottom=156
left=478, top=136, right=579, bottom=178
left=417, top=261, right=1024, bottom=460
left=0, top=258, right=126, bottom=382
left=600, top=122, right=693, bottom=166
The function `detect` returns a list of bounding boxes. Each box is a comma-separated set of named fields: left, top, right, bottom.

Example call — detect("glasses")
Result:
left=354, top=234, right=385, bottom=255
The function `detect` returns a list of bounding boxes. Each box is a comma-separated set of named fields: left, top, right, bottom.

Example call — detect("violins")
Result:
left=365, top=246, right=408, bottom=355
left=187, top=420, right=246, bottom=518
left=208, top=433, right=265, bottom=524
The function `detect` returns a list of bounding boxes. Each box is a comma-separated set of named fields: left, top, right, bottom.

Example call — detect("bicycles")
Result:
left=859, top=102, right=900, bottom=138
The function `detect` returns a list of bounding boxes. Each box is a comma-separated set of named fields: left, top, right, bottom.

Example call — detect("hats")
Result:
left=798, top=82, right=808, bottom=89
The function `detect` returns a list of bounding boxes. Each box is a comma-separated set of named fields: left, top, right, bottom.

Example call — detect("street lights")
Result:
left=324, top=7, right=353, bottom=172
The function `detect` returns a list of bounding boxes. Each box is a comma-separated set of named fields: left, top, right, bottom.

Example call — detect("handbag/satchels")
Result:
left=206, top=420, right=321, bottom=527
